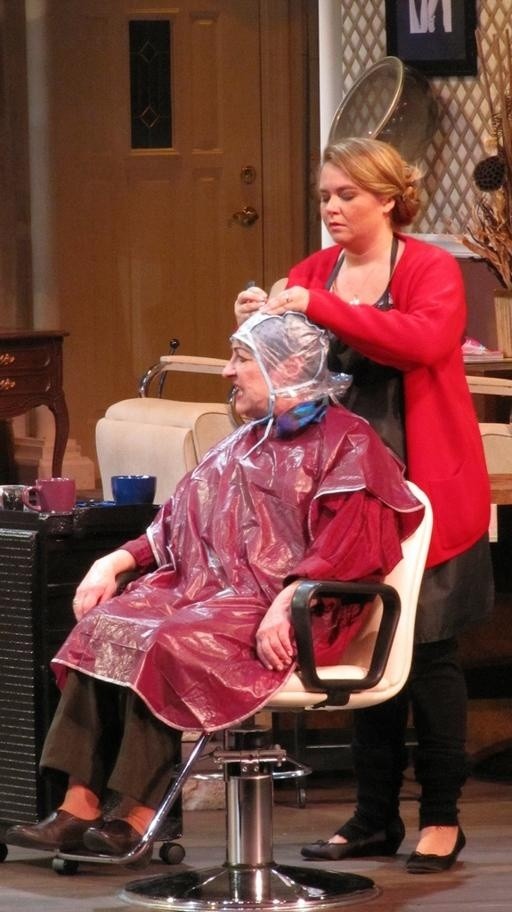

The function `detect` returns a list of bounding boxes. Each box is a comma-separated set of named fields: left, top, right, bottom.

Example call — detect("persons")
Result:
left=233, top=135, right=497, bottom=873
left=3, top=312, right=425, bottom=853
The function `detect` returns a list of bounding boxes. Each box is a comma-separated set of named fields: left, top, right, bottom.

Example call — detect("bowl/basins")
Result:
left=328, top=55, right=445, bottom=163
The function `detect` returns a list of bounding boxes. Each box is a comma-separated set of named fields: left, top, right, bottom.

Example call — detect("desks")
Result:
left=462, top=356, right=511, bottom=424
left=0, top=327, right=71, bottom=477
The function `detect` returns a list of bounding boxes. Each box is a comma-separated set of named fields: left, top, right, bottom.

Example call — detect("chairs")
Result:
left=56, top=481, right=433, bottom=912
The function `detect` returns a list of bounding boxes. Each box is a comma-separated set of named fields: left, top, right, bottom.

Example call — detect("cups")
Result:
left=17, top=476, right=75, bottom=516
left=109, top=473, right=159, bottom=505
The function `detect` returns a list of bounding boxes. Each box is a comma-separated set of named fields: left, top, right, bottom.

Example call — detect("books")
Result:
left=461, top=335, right=503, bottom=365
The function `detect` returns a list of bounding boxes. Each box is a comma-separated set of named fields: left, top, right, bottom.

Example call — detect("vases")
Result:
left=492, top=289, right=512, bottom=358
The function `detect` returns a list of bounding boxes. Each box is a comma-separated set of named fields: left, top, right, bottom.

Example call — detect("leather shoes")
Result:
left=301, top=815, right=405, bottom=860
left=82, top=819, right=153, bottom=870
left=6, top=809, right=105, bottom=850
left=406, top=824, right=465, bottom=874
